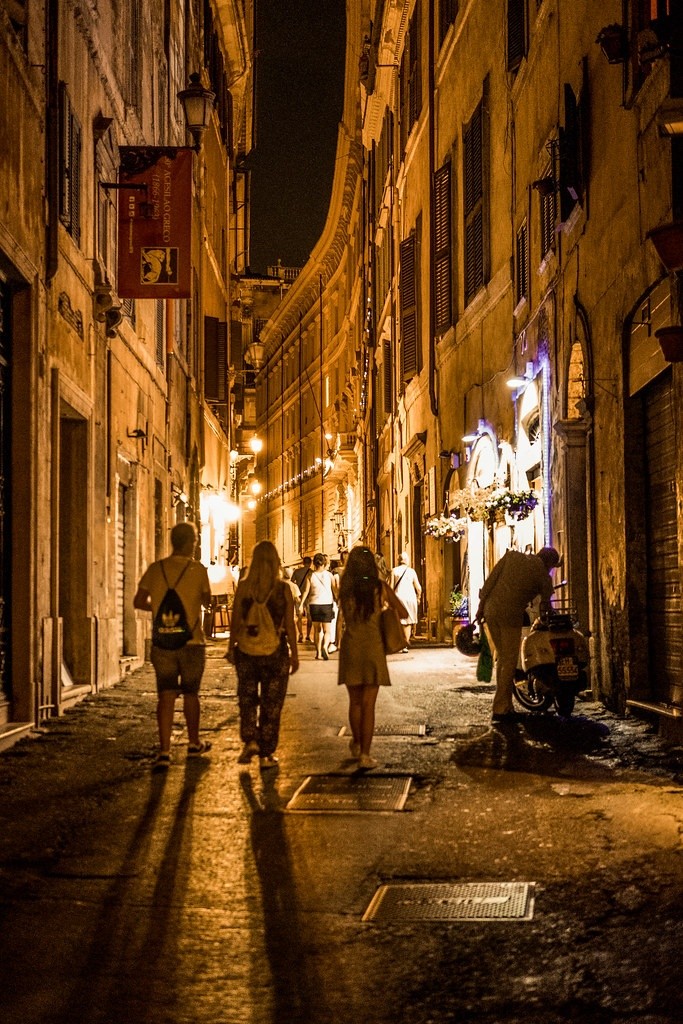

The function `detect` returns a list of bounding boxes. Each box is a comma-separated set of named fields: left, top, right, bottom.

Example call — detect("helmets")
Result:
left=455, top=624, right=482, bottom=657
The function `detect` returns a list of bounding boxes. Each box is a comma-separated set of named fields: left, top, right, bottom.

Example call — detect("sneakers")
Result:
left=237, top=738, right=260, bottom=765
left=491, top=706, right=527, bottom=724
left=349, top=737, right=361, bottom=758
left=187, top=740, right=213, bottom=757
left=359, top=752, right=379, bottom=770
left=261, top=754, right=281, bottom=770
left=149, top=749, right=172, bottom=771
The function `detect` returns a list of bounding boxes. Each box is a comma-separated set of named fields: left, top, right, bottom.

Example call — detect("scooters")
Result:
left=511, top=580, right=591, bottom=718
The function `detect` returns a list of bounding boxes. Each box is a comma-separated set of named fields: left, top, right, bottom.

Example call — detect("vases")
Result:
left=452, top=617, right=470, bottom=645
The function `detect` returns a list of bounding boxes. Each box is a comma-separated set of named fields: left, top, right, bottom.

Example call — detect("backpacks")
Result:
left=152, top=559, right=194, bottom=652
left=235, top=581, right=285, bottom=657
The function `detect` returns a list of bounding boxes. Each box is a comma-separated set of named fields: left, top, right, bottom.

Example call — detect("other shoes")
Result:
left=297, top=634, right=304, bottom=643
left=305, top=635, right=314, bottom=643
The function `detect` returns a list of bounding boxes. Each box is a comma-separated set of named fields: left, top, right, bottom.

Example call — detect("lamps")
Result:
left=229, top=437, right=264, bottom=465
left=235, top=336, right=265, bottom=375
left=177, top=71, right=215, bottom=156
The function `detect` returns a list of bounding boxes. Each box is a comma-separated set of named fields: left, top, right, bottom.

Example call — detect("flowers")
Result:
left=422, top=515, right=467, bottom=543
left=486, top=486, right=538, bottom=521
left=450, top=488, right=492, bottom=522
left=450, top=584, right=469, bottom=616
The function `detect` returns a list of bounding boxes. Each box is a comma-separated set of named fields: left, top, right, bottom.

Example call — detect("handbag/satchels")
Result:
left=381, top=604, right=408, bottom=655
left=476, top=625, right=494, bottom=684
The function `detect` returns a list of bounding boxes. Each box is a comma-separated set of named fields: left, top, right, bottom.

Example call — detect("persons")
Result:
left=336, top=546, right=408, bottom=768
left=476, top=546, right=559, bottom=720
left=373, top=552, right=390, bottom=585
left=227, top=540, right=299, bottom=768
left=328, top=552, right=351, bottom=653
left=292, top=557, right=314, bottom=642
left=388, top=553, right=422, bottom=653
left=134, top=523, right=212, bottom=770
left=298, top=553, right=339, bottom=659
